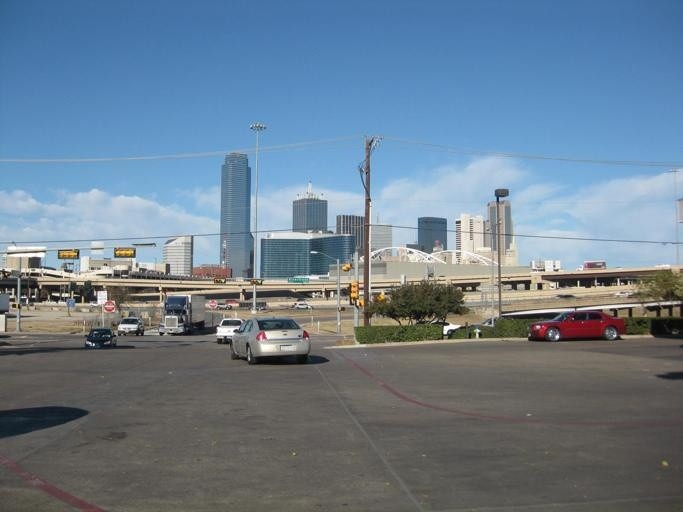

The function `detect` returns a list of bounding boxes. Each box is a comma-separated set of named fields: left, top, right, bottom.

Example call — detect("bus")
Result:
left=239, top=301, right=269, bottom=309
left=582, top=261, right=606, bottom=270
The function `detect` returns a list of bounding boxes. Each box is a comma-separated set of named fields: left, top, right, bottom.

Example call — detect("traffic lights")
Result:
left=351, top=281, right=359, bottom=300
left=343, top=263, right=350, bottom=271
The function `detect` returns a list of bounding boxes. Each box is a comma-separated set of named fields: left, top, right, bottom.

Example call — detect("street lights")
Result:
left=494, top=188, right=509, bottom=318
left=249, top=123, right=267, bottom=313
left=309, top=250, right=342, bottom=334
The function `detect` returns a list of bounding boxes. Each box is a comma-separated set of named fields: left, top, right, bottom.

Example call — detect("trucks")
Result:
left=158, top=294, right=206, bottom=335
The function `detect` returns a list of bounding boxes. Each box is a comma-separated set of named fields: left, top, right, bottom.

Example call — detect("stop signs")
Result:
left=209, top=300, right=217, bottom=309
left=103, top=300, right=116, bottom=313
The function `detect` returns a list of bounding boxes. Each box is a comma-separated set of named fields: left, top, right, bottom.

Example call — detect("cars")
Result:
left=216, top=316, right=311, bottom=364
left=216, top=303, right=232, bottom=311
left=294, top=302, right=313, bottom=309
left=416, top=317, right=462, bottom=337
left=84, top=330, right=118, bottom=349
left=117, top=317, right=146, bottom=337
left=529, top=310, right=626, bottom=341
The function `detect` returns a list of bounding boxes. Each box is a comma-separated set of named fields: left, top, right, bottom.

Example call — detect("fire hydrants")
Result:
left=474, top=328, right=481, bottom=339
